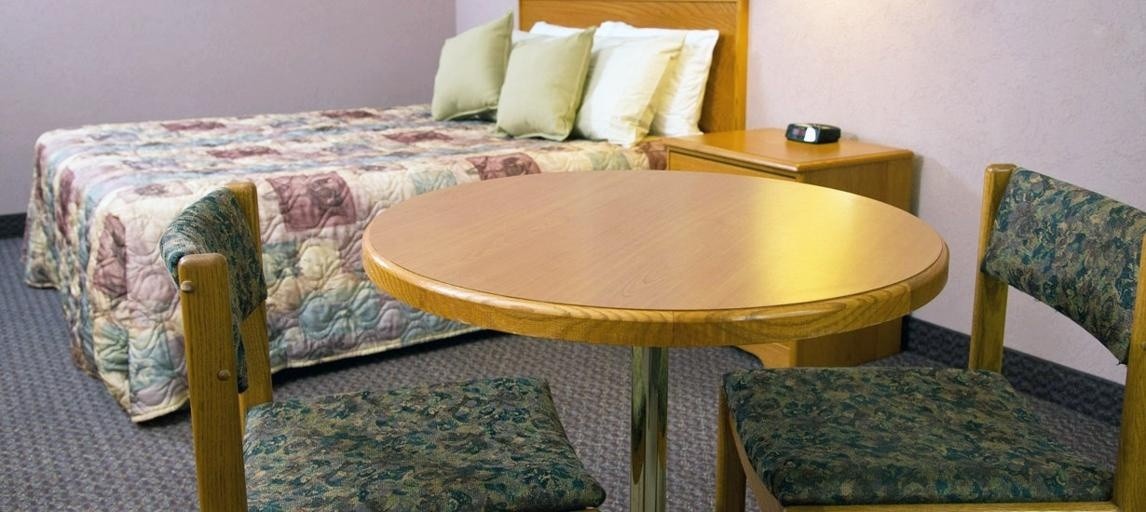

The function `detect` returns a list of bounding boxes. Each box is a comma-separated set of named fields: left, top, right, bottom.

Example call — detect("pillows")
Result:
left=430, top=9, right=596, bottom=142
left=592, top=20, right=719, bottom=139
left=571, top=34, right=686, bottom=149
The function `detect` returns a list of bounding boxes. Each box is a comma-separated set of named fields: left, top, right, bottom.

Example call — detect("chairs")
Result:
left=713, top=163, right=1145, bottom=511
left=158, top=181, right=607, bottom=512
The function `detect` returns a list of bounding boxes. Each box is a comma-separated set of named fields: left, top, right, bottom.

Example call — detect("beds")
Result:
left=20, top=1, right=749, bottom=424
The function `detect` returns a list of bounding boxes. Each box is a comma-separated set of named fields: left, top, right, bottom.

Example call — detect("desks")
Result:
left=361, top=168, right=949, bottom=512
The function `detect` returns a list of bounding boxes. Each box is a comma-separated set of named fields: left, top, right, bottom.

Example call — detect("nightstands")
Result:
left=661, top=127, right=914, bottom=371
